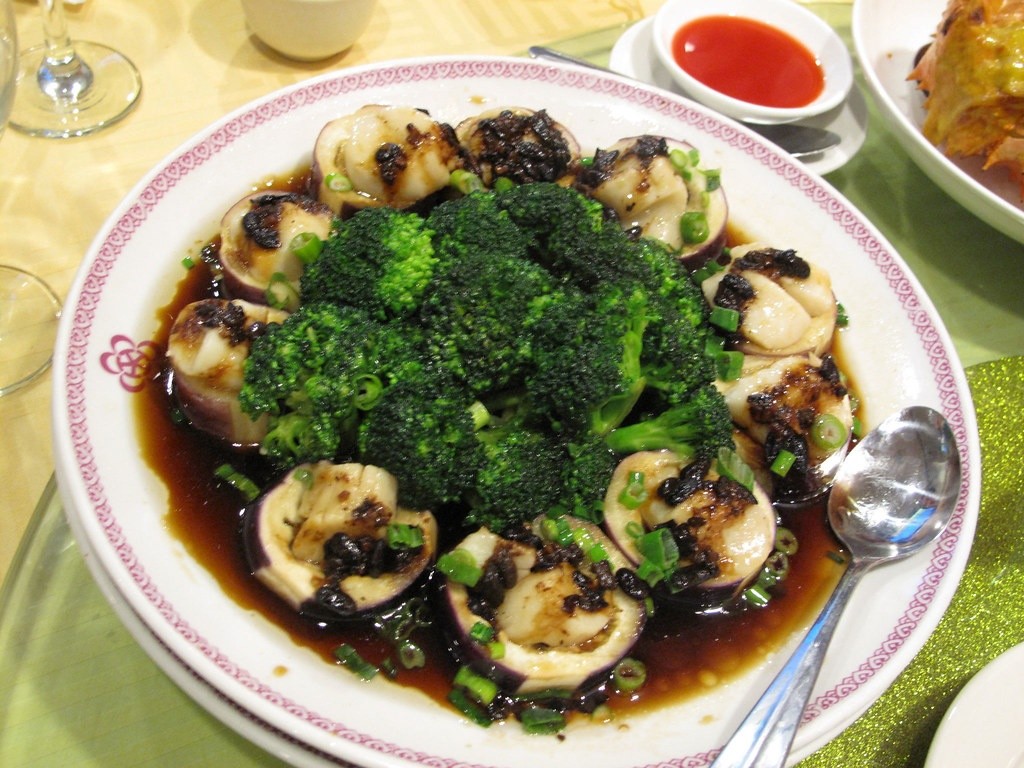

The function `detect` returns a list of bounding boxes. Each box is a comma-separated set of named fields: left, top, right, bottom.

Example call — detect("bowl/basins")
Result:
left=241, top=0, right=378, bottom=62
left=654, top=0, right=853, bottom=124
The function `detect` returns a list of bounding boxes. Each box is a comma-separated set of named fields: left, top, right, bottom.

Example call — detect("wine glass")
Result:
left=0, top=0, right=62, bottom=397
left=7, top=0, right=143, bottom=139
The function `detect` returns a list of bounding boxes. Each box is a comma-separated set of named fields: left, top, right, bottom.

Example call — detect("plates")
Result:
left=849, top=0, right=1024, bottom=244
left=49, top=58, right=980, bottom=768
left=922, top=641, right=1024, bottom=768
left=609, top=13, right=868, bottom=175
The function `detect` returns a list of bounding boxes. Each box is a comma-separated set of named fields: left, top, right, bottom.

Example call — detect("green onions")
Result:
left=165, top=142, right=865, bottom=732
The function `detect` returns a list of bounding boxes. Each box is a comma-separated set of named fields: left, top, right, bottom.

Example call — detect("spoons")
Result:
left=528, top=46, right=841, bottom=157
left=710, top=406, right=961, bottom=768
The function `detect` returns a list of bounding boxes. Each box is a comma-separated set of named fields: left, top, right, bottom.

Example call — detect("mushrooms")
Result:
left=164, top=98, right=848, bottom=696
